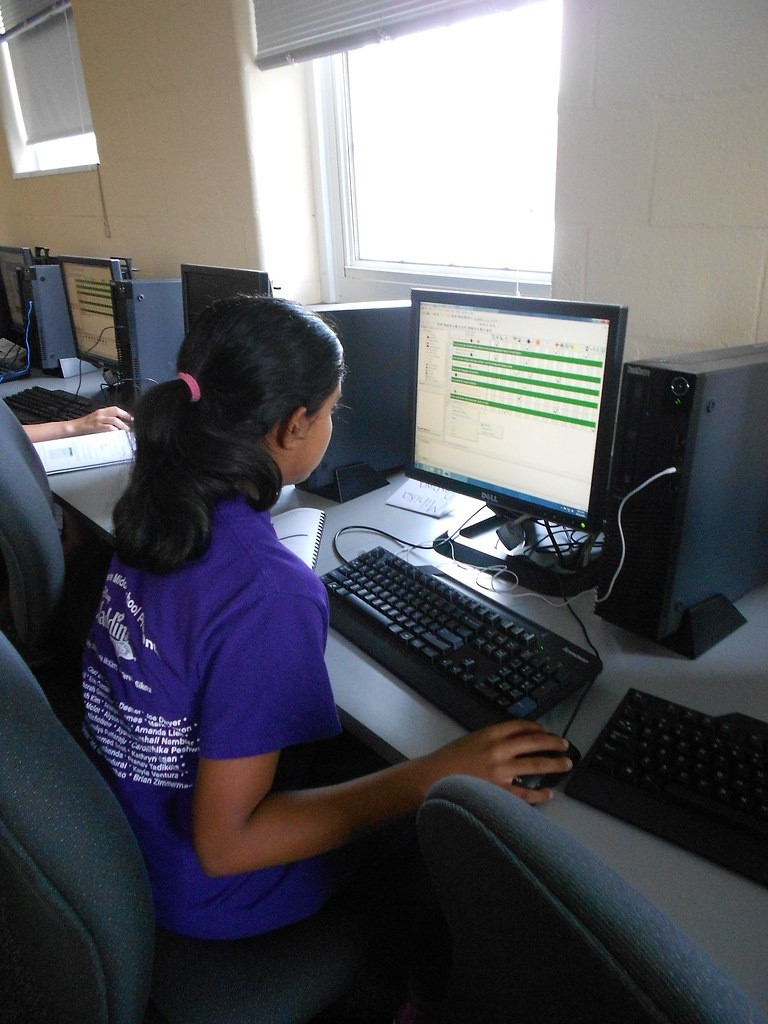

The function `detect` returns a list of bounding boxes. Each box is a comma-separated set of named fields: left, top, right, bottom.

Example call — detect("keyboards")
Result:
left=2, top=385, right=106, bottom=425
left=563, top=688, right=768, bottom=890
left=318, top=544, right=604, bottom=735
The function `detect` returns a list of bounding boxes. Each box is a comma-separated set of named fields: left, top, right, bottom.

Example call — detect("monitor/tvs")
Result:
left=404, top=288, right=628, bottom=598
left=0, top=246, right=35, bottom=350
left=57, top=255, right=124, bottom=406
left=179, top=264, right=274, bottom=339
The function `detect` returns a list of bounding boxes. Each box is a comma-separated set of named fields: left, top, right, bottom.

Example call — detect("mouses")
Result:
left=511, top=734, right=582, bottom=808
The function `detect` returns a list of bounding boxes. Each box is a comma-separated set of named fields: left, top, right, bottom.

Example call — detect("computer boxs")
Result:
left=295, top=299, right=413, bottom=503
left=15, top=265, right=77, bottom=374
left=107, top=278, right=185, bottom=412
left=593, top=342, right=768, bottom=643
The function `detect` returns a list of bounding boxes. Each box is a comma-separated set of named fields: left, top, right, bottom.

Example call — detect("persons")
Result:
left=22, top=406, right=135, bottom=443
left=81, top=295, right=572, bottom=943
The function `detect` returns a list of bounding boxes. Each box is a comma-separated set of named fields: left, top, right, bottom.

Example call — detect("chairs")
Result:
left=0, top=629, right=425, bottom=1024
left=416, top=775, right=768, bottom=1024
left=0, top=396, right=110, bottom=732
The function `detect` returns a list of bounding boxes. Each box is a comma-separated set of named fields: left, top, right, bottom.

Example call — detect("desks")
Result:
left=0, top=338, right=768, bottom=1024
